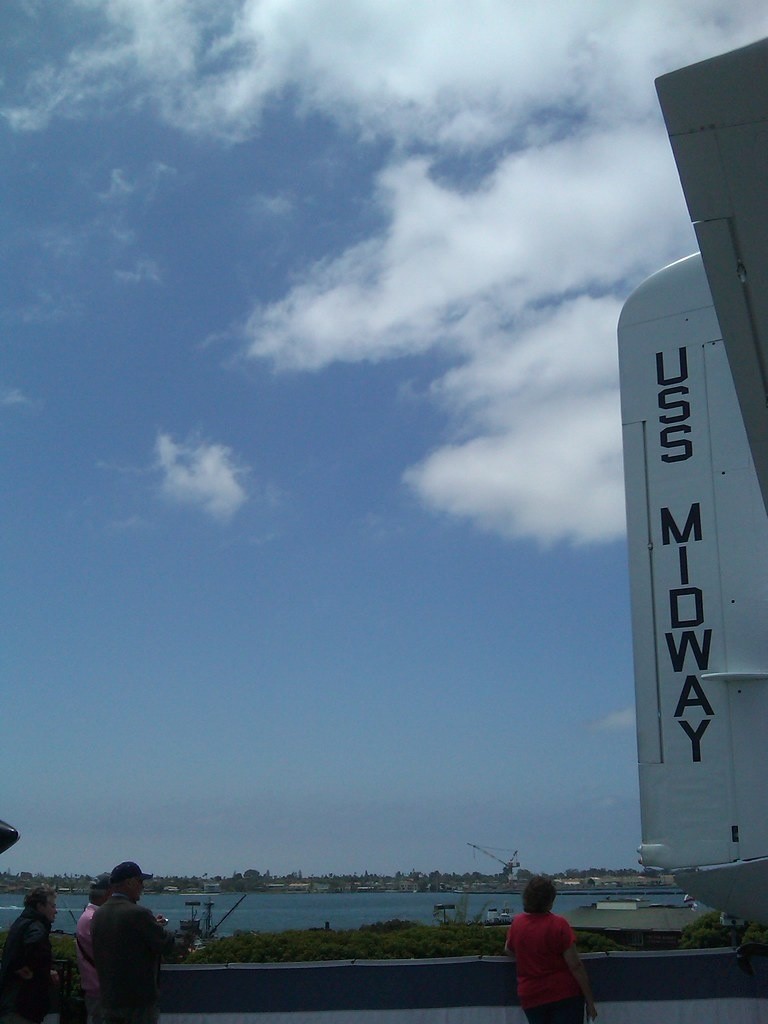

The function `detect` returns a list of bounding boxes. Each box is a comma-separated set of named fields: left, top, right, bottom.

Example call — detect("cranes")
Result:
left=466, top=842, right=521, bottom=870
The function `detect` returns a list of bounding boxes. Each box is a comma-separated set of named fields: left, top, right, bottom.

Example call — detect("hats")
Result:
left=110, top=862, right=153, bottom=884
left=89, top=872, right=111, bottom=889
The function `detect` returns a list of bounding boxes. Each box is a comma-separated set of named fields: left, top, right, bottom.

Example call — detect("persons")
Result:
left=0, top=884, right=59, bottom=1024
left=504, top=876, right=599, bottom=1024
left=73, top=862, right=171, bottom=1024
